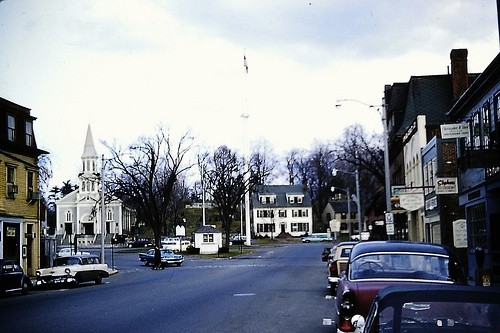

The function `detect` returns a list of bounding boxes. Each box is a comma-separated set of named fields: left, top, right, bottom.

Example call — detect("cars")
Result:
left=350, top=283, right=500, bottom=333
left=127, top=237, right=155, bottom=248
left=300, top=233, right=327, bottom=243
left=0, top=259, right=32, bottom=298
left=322, top=242, right=386, bottom=291
left=56, top=247, right=90, bottom=257
left=138, top=249, right=185, bottom=267
left=334, top=240, right=467, bottom=333
left=229, top=235, right=256, bottom=246
left=36, top=254, right=118, bottom=290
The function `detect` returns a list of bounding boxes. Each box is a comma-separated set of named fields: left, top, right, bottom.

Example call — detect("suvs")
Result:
left=351, top=232, right=370, bottom=241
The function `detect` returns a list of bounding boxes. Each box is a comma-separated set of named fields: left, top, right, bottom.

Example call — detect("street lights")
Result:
left=99, top=152, right=134, bottom=264
left=331, top=186, right=351, bottom=241
left=332, top=167, right=362, bottom=243
left=335, top=98, right=395, bottom=267
left=201, top=162, right=220, bottom=226
